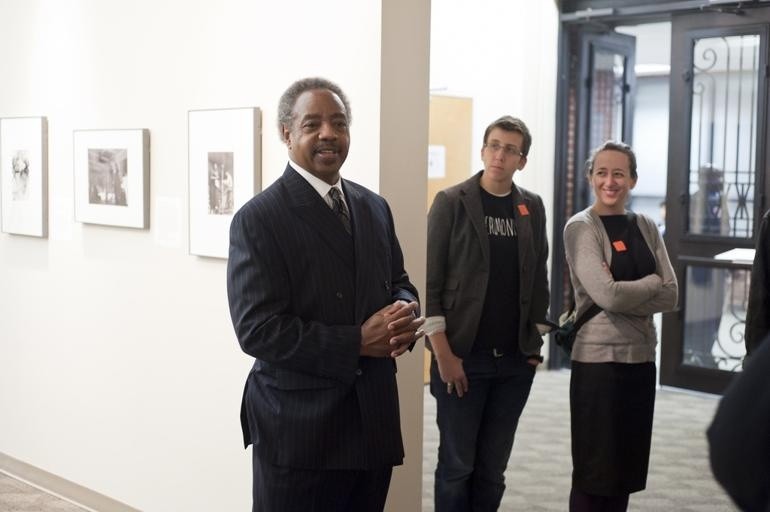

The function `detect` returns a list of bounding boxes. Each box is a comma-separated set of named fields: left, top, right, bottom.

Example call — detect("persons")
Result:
left=210, top=160, right=233, bottom=215
left=744, top=209, right=770, bottom=365
left=657, top=202, right=665, bottom=235
left=685, top=163, right=737, bottom=371
left=705, top=334, right=769, bottom=511
left=562, top=139, right=680, bottom=511
left=226, top=76, right=426, bottom=512
left=426, top=115, right=562, bottom=512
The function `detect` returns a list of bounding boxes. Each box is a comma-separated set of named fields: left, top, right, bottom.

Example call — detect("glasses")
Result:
left=483, top=142, right=525, bottom=157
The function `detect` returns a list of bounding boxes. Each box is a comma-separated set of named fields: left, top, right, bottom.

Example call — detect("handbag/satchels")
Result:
left=555, top=309, right=578, bottom=357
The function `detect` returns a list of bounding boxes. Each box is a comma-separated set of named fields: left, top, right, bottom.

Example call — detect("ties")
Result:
left=328, top=187, right=353, bottom=238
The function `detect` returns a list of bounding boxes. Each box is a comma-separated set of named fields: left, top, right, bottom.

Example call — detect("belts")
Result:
left=478, top=342, right=518, bottom=358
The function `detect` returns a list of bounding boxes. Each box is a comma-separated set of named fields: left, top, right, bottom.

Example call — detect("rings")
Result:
left=447, top=381, right=453, bottom=388
